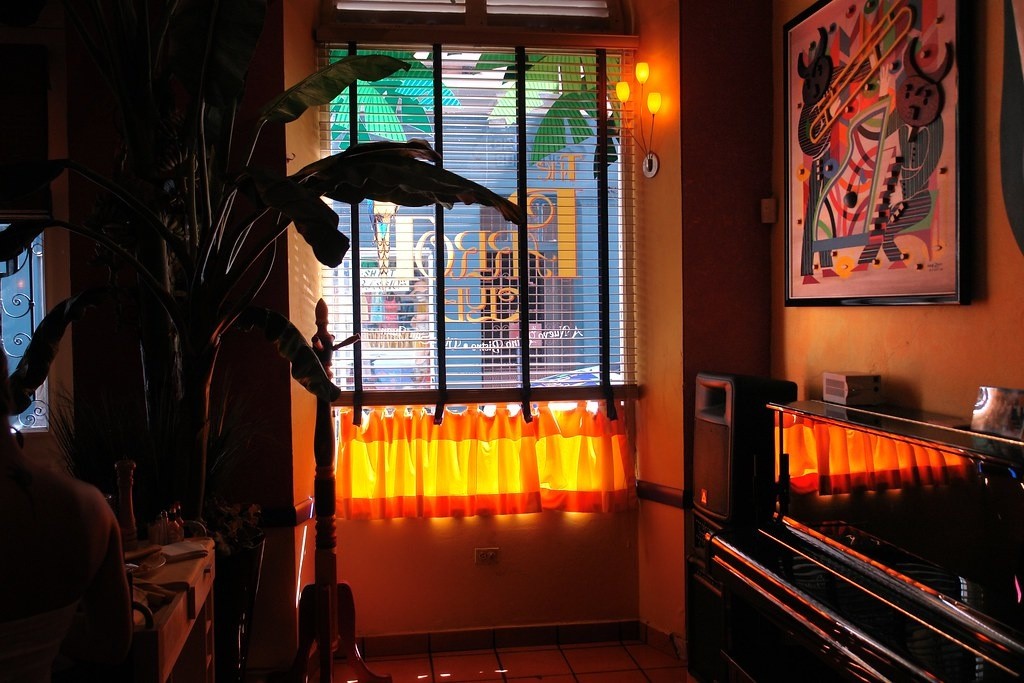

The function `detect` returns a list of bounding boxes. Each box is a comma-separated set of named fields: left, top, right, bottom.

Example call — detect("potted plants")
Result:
left=0, top=0, right=524, bottom=682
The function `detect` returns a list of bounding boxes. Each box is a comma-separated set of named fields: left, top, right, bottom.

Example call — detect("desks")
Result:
left=121, top=539, right=217, bottom=683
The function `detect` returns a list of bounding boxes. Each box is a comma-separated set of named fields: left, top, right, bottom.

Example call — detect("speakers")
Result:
left=693, top=372, right=798, bottom=528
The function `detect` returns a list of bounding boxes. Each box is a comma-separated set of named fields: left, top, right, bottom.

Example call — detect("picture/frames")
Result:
left=781, top=0, right=975, bottom=307
left=969, top=383, right=1024, bottom=440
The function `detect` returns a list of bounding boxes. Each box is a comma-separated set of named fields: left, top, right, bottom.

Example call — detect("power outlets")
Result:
left=475, top=547, right=500, bottom=565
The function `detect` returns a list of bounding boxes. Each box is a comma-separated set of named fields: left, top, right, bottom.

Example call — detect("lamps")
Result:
left=615, top=62, right=662, bottom=178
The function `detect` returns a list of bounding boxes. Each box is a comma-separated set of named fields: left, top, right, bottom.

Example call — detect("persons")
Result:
left=0, top=353, right=133, bottom=683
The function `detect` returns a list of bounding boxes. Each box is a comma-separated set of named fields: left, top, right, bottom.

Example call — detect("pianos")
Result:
left=704, top=396, right=1023, bottom=683
